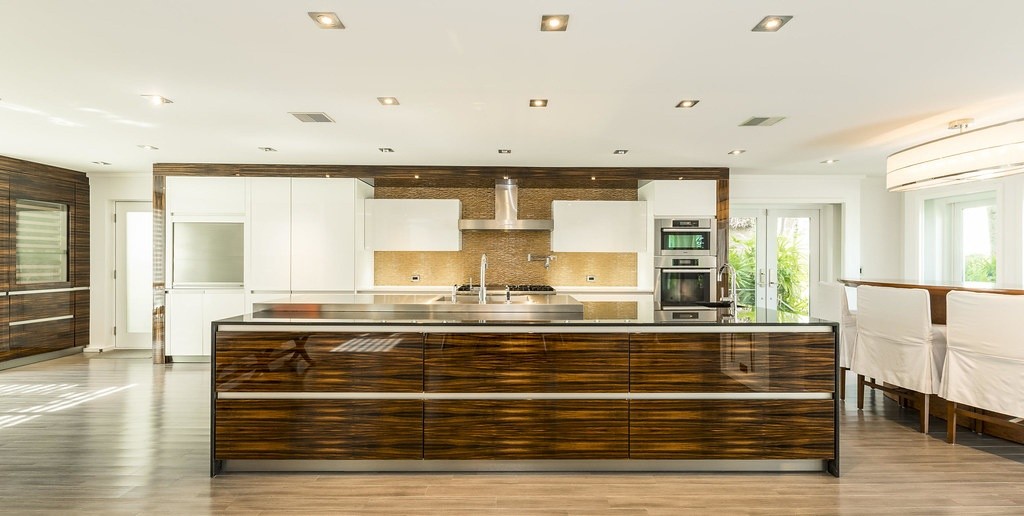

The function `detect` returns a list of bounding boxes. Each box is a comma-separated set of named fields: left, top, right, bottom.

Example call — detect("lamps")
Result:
left=886, top=117, right=1024, bottom=192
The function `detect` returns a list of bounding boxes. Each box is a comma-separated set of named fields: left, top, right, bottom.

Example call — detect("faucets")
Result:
left=478, top=253, right=489, bottom=304
left=717, top=263, right=736, bottom=318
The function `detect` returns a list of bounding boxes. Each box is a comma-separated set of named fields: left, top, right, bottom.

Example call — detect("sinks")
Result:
left=437, top=295, right=529, bottom=301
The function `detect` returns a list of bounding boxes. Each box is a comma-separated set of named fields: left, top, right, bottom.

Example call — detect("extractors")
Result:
left=458, top=178, right=553, bottom=231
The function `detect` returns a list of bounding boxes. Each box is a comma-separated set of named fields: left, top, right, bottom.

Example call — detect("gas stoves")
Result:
left=455, top=284, right=556, bottom=295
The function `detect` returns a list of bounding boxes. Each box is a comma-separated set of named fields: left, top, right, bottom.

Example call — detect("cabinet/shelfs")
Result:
left=422, top=334, right=630, bottom=462
left=629, top=333, right=835, bottom=461
left=550, top=199, right=647, bottom=254
left=164, top=289, right=246, bottom=357
left=363, top=197, right=463, bottom=252
left=213, top=330, right=422, bottom=461
left=249, top=175, right=373, bottom=295
left=166, top=176, right=248, bottom=217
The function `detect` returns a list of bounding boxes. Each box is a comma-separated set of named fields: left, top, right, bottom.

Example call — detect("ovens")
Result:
left=654, top=269, right=717, bottom=321
left=654, top=216, right=717, bottom=268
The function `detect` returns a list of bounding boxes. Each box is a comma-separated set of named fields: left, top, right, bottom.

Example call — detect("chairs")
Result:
left=850, top=285, right=977, bottom=436
left=937, top=291, right=1024, bottom=446
left=809, top=280, right=875, bottom=402
